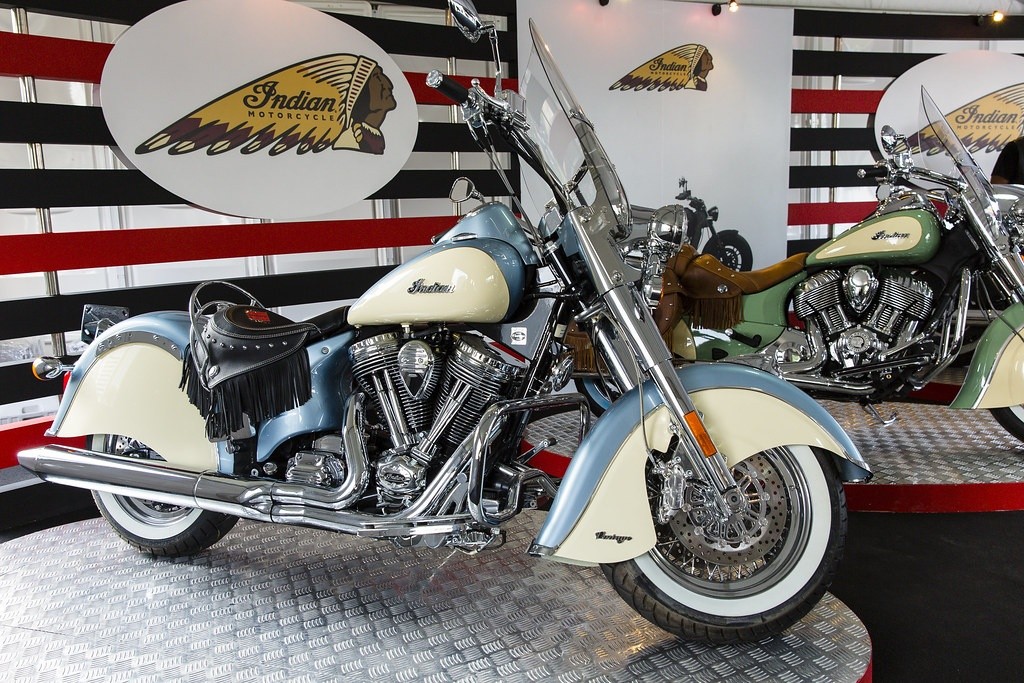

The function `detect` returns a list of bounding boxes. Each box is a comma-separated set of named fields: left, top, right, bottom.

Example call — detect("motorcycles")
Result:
left=16, top=1, right=874, bottom=645
left=565, top=85, right=1024, bottom=448
left=628, top=177, right=754, bottom=276
